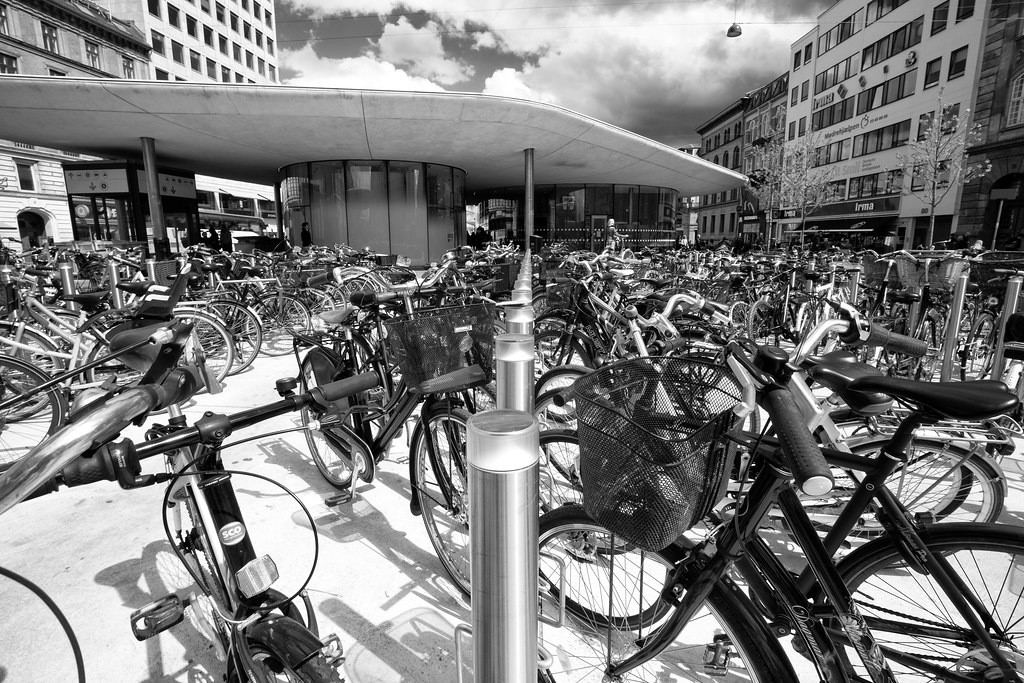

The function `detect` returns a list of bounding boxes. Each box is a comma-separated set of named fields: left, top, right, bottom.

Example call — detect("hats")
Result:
left=607, top=219, right=615, bottom=227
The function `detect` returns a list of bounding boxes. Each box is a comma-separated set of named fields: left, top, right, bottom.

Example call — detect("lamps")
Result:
left=727, top=23, right=743, bottom=38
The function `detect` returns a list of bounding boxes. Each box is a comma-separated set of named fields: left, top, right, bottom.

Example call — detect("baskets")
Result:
left=867, top=248, right=1024, bottom=296
left=0, top=279, right=18, bottom=316
left=382, top=303, right=495, bottom=396
left=155, top=260, right=177, bottom=287
left=572, top=354, right=750, bottom=554
left=539, top=256, right=574, bottom=311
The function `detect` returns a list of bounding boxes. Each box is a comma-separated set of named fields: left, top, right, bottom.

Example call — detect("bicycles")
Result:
left=0, top=372, right=382, bottom=683
left=0, top=234, right=1023, bottom=683
left=522, top=357, right=1017, bottom=680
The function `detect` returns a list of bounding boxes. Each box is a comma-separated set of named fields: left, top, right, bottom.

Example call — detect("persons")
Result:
left=467, top=226, right=491, bottom=250
left=507, top=229, right=513, bottom=239
left=604, top=218, right=629, bottom=250
left=218, top=224, right=232, bottom=253
left=301, top=222, right=312, bottom=247
left=206, top=224, right=220, bottom=251
